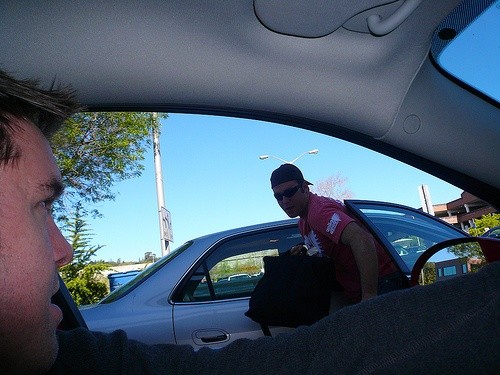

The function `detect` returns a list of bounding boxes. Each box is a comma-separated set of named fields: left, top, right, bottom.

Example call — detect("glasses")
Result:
left=274, top=182, right=302, bottom=201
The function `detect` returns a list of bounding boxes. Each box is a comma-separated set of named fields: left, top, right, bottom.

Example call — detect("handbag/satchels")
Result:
left=243, top=241, right=335, bottom=337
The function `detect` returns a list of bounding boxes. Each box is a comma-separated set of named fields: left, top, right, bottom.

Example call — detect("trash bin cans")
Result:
left=108, top=271, right=143, bottom=294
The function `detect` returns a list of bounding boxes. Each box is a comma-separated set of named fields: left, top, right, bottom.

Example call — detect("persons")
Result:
left=270, top=163, right=408, bottom=316
left=0, top=69, right=500, bottom=375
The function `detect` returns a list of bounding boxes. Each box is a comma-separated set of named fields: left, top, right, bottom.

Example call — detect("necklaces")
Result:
left=301, top=217, right=319, bottom=255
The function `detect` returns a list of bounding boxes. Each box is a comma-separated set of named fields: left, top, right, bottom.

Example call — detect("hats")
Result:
left=270, top=163, right=314, bottom=189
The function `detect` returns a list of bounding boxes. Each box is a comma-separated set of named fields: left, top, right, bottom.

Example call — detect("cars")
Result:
left=77, top=199, right=482, bottom=350
